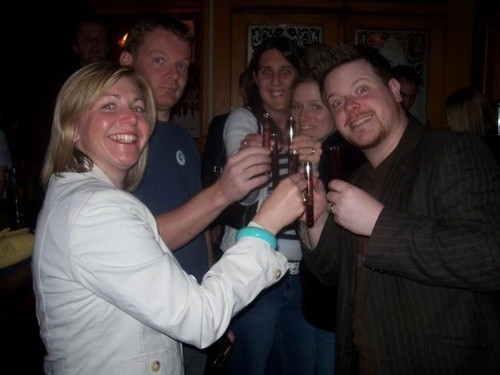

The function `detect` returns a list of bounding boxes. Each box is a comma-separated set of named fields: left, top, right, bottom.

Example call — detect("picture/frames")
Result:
left=245, top=22, right=324, bottom=68
left=352, top=26, right=433, bottom=127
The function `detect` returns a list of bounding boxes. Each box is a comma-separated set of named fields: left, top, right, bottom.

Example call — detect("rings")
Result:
left=241, top=139, right=247, bottom=147
left=303, top=194, right=306, bottom=204
left=329, top=204, right=334, bottom=215
left=310, top=147, right=315, bottom=154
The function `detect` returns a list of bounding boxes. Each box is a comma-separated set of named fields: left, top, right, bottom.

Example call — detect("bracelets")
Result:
left=236, top=227, right=278, bottom=250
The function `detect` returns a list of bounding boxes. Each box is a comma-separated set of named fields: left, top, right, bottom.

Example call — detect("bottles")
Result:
left=5, top=166, right=26, bottom=230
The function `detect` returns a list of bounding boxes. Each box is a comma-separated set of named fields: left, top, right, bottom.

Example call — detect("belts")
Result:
left=287, top=262, right=301, bottom=275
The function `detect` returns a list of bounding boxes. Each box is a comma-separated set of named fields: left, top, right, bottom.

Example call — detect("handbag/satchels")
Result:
left=202, top=106, right=256, bottom=231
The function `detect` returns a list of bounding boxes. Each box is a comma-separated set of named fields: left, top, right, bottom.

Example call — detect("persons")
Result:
left=0, top=132, right=14, bottom=231
left=31, top=61, right=308, bottom=375
left=71, top=14, right=500, bottom=375
